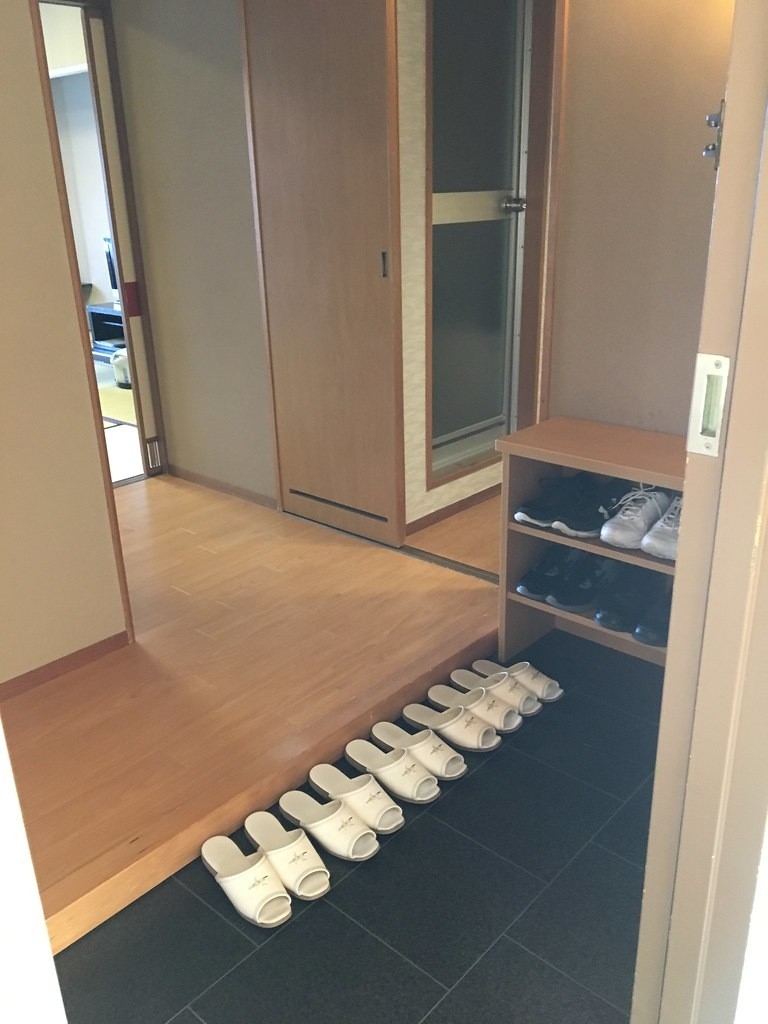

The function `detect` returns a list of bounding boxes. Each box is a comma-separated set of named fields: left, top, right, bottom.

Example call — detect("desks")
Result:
left=98, top=385, right=137, bottom=428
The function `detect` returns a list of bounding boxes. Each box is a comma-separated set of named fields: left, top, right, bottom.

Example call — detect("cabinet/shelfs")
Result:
left=495, top=416, right=686, bottom=667
left=86, top=302, right=125, bottom=355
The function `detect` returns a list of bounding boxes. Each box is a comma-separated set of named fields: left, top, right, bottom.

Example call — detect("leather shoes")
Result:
left=595, top=565, right=673, bottom=647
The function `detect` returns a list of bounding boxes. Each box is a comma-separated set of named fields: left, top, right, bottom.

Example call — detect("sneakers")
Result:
left=515, top=542, right=621, bottom=611
left=600, top=487, right=683, bottom=561
left=515, top=468, right=631, bottom=538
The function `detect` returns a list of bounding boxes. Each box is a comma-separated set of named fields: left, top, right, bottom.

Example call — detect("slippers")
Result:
left=402, top=683, right=524, bottom=753
left=345, top=720, right=470, bottom=805
left=276, top=761, right=407, bottom=861
left=451, top=659, right=567, bottom=717
left=199, top=811, right=332, bottom=929
